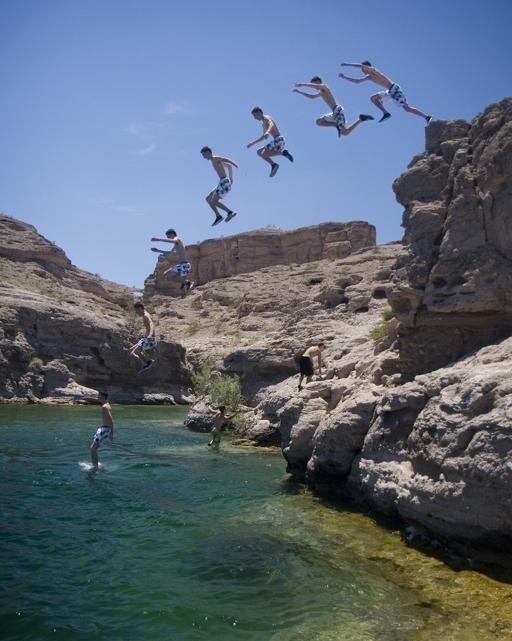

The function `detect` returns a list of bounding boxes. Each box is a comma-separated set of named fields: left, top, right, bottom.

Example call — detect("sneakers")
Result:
left=269, top=149, right=294, bottom=178
left=180, top=280, right=195, bottom=290
left=212, top=211, right=238, bottom=226
left=336, top=112, right=433, bottom=139
left=137, top=359, right=156, bottom=374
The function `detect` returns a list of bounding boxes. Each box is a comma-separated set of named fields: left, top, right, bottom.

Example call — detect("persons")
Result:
left=208, top=404, right=241, bottom=448
left=293, top=76, right=374, bottom=139
left=247, top=107, right=293, bottom=177
left=90, top=390, right=113, bottom=466
left=338, top=61, right=434, bottom=124
left=129, top=302, right=157, bottom=375
left=298, top=343, right=324, bottom=388
left=199, top=147, right=239, bottom=227
left=150, top=228, right=195, bottom=293
